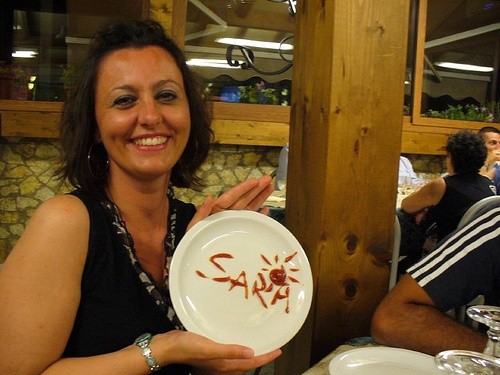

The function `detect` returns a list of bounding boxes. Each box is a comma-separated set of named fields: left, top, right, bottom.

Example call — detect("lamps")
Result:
left=187, top=58, right=245, bottom=69
left=431, top=60, right=493, bottom=73
left=215, top=36, right=294, bottom=51
left=12, top=49, right=39, bottom=59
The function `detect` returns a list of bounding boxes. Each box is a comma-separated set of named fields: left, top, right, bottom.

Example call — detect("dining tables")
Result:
left=260, top=186, right=410, bottom=211
left=300, top=336, right=395, bottom=375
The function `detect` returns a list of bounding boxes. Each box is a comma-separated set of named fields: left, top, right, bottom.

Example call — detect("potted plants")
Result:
left=0, top=66, right=40, bottom=101
left=58, top=58, right=88, bottom=99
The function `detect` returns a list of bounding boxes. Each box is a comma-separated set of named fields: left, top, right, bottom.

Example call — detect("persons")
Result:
left=477, top=126, right=500, bottom=195
left=371, top=198, right=500, bottom=357
left=396, top=156, right=433, bottom=280
left=401, top=126, right=500, bottom=306
left=0, top=17, right=282, bottom=375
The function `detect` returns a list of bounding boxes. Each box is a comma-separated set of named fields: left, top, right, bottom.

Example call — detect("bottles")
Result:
left=434, top=349, right=500, bottom=375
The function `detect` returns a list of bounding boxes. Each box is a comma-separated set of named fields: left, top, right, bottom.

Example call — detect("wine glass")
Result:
left=467, top=305, right=500, bottom=356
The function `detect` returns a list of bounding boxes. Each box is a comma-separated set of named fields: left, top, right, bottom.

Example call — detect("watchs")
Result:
left=135, top=333, right=160, bottom=372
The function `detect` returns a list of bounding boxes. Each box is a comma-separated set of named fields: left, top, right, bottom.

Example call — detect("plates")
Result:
left=329, top=347, right=442, bottom=375
left=169, top=210, right=314, bottom=361
